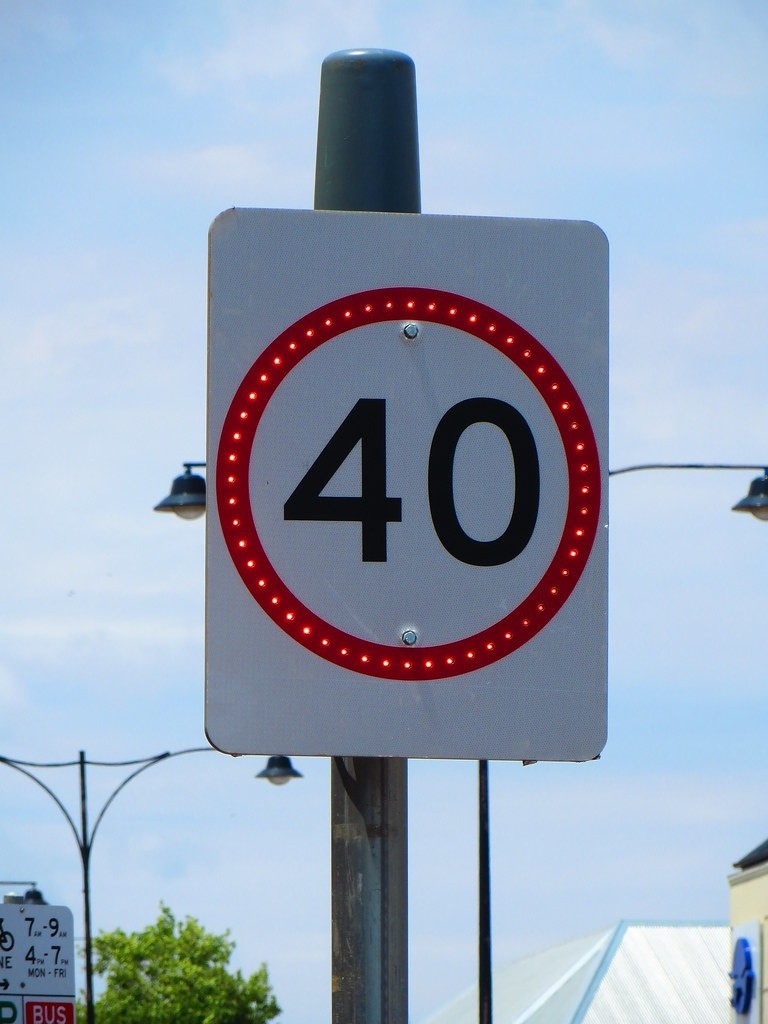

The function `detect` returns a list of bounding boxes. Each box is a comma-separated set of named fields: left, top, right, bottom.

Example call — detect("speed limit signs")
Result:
left=197, top=199, right=611, bottom=763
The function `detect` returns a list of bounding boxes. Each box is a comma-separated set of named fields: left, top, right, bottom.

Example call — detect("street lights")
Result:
left=150, top=462, right=768, bottom=1024
left=0, top=739, right=305, bottom=1024
